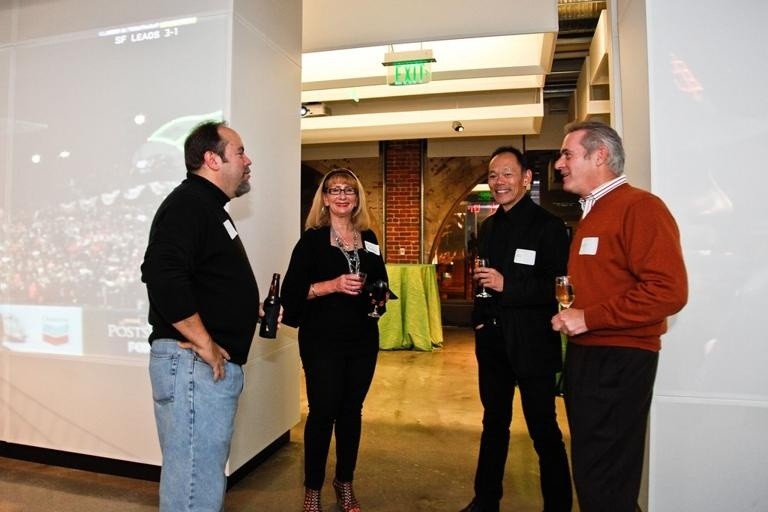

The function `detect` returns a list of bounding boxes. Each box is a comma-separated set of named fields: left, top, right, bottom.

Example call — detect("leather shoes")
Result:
left=458, top=502, right=500, bottom=512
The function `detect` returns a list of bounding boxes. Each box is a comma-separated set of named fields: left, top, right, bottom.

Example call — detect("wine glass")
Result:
left=368, top=281, right=387, bottom=319
left=555, top=275, right=576, bottom=308
left=474, top=258, right=493, bottom=299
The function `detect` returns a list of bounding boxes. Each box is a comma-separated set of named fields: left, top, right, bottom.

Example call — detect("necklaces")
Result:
left=332, top=228, right=363, bottom=295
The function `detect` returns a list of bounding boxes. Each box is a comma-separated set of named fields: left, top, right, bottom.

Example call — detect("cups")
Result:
left=350, top=270, right=367, bottom=293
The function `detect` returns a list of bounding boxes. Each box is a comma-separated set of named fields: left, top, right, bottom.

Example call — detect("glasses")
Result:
left=322, top=188, right=358, bottom=195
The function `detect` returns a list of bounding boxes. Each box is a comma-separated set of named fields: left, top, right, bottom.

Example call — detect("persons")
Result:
left=280, top=167, right=389, bottom=511
left=140, top=118, right=259, bottom=511
left=461, top=145, right=573, bottom=512
left=550, top=121, right=689, bottom=512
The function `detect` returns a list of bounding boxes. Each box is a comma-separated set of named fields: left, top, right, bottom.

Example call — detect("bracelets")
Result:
left=310, top=283, right=318, bottom=298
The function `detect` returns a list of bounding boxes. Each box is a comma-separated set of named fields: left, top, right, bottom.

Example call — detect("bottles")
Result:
left=259, top=273, right=282, bottom=339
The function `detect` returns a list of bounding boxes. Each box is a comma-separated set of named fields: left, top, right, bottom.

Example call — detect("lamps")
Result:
left=451, top=120, right=465, bottom=134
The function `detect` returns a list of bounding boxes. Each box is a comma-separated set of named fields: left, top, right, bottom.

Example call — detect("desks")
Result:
left=371, top=259, right=444, bottom=353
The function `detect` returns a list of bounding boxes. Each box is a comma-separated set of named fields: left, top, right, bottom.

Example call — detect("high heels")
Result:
left=303, top=486, right=323, bottom=512
left=332, top=476, right=360, bottom=512
